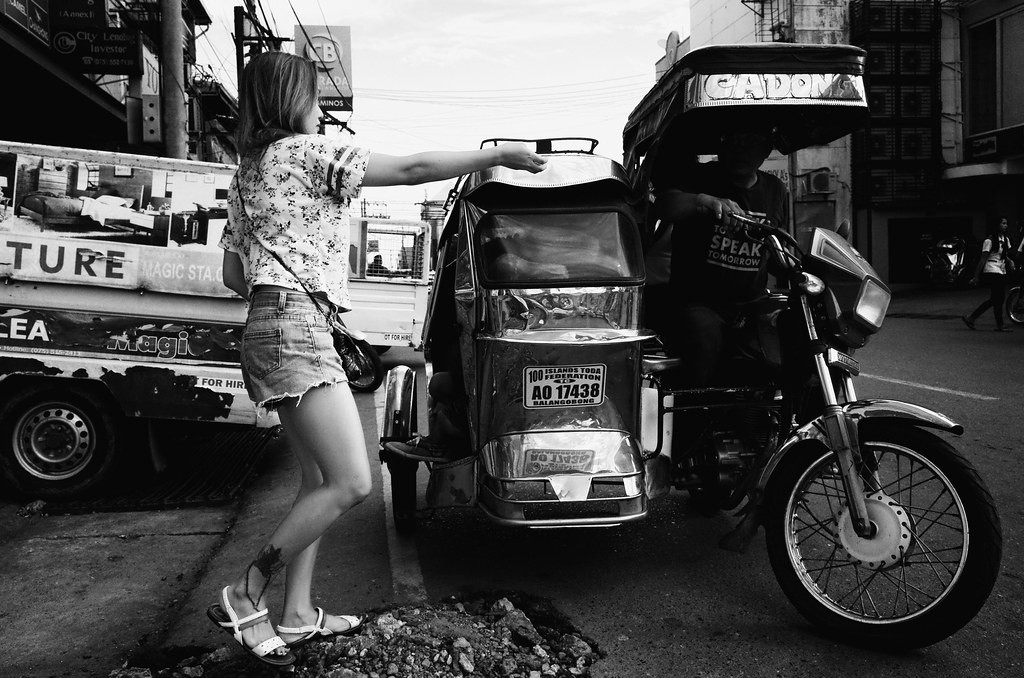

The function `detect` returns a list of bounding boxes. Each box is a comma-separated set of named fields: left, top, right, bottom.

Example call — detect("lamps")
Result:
left=855, top=127, right=934, bottom=161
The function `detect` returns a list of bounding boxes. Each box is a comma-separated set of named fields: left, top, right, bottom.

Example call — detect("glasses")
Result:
left=721, top=128, right=768, bottom=149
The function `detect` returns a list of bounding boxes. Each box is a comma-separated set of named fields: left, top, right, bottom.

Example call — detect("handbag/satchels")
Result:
left=329, top=325, right=371, bottom=380
left=1005, top=257, right=1016, bottom=273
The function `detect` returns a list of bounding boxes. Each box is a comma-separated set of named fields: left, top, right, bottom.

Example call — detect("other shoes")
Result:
left=998, top=324, right=1014, bottom=332
left=962, top=312, right=977, bottom=330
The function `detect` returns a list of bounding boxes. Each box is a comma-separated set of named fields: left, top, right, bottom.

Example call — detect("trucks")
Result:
left=0, top=138, right=286, bottom=506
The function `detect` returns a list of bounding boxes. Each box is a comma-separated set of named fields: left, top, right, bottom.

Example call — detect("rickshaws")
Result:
left=373, top=41, right=1007, bottom=658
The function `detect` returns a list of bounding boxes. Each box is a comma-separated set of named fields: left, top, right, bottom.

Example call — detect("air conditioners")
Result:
left=859, top=44, right=934, bottom=75
left=807, top=171, right=838, bottom=193
left=855, top=0, right=935, bottom=32
left=859, top=170, right=933, bottom=207
left=866, top=86, right=933, bottom=117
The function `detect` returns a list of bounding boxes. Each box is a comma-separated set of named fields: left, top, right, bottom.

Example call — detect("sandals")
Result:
left=206, top=585, right=296, bottom=667
left=276, top=606, right=368, bottom=648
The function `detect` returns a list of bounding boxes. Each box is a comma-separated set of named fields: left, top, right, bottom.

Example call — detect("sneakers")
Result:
left=713, top=483, right=750, bottom=516
left=384, top=431, right=448, bottom=463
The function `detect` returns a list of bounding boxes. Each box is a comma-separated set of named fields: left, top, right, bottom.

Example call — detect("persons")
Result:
left=962, top=216, right=1015, bottom=331
left=367, top=255, right=391, bottom=277
left=651, top=119, right=797, bottom=514
left=207, top=53, right=548, bottom=666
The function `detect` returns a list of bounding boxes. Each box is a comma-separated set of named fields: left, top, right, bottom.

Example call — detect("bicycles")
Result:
left=1005, top=258, right=1024, bottom=326
